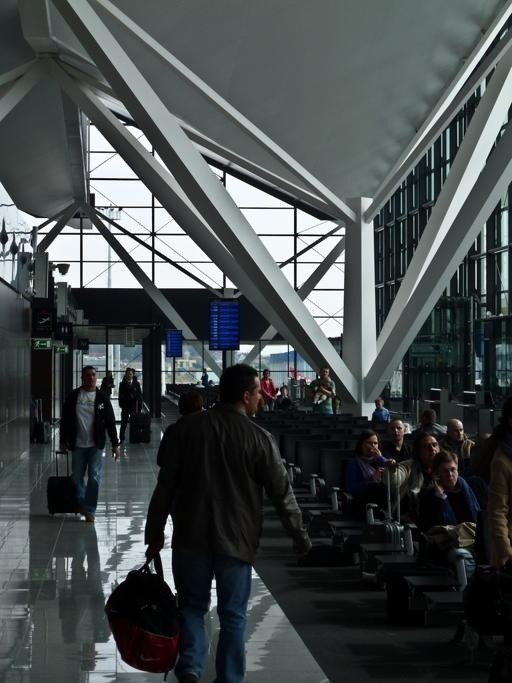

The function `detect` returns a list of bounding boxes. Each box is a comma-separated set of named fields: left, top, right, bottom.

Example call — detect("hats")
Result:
left=431, top=451, right=460, bottom=474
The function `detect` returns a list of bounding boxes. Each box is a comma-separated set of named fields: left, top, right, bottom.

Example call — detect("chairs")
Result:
left=166, top=381, right=429, bottom=537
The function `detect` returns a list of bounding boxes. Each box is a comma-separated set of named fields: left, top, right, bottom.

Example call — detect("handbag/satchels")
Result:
left=102, top=570, right=182, bottom=673
left=462, top=569, right=511, bottom=635
left=425, top=522, right=479, bottom=548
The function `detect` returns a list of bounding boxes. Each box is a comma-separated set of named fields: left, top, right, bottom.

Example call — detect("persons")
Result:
left=60, top=366, right=214, bottom=522
left=256, top=365, right=337, bottom=413
left=143, top=364, right=312, bottom=683
left=350, top=394, right=512, bottom=679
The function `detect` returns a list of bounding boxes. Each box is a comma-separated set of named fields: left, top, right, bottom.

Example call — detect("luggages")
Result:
left=369, top=463, right=406, bottom=582
left=129, top=395, right=150, bottom=443
left=47, top=451, right=80, bottom=513
